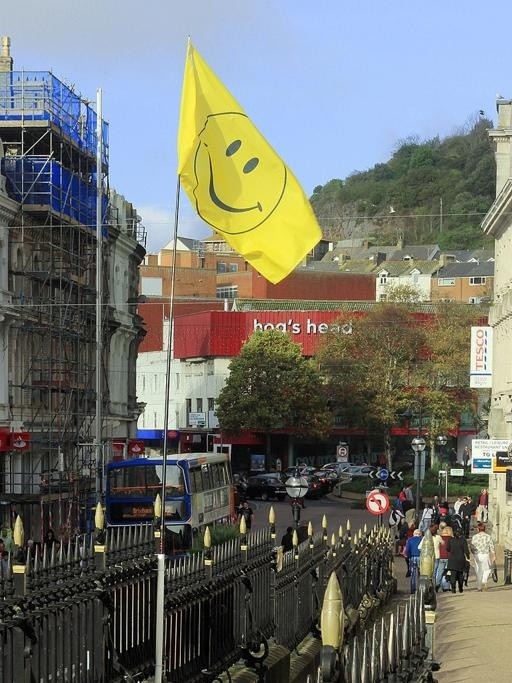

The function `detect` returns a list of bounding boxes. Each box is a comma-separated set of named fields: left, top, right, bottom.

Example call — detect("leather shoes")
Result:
left=473, top=583, right=488, bottom=592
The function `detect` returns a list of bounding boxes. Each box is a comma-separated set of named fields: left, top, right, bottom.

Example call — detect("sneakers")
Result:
left=406, top=569, right=411, bottom=577
left=435, top=581, right=468, bottom=593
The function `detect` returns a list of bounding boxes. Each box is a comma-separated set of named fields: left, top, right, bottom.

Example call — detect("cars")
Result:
left=232, top=459, right=411, bottom=512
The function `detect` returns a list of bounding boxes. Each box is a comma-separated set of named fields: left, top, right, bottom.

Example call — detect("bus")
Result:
left=105, top=451, right=238, bottom=555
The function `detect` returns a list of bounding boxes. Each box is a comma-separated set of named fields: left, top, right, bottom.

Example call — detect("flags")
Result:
left=176, top=33, right=323, bottom=287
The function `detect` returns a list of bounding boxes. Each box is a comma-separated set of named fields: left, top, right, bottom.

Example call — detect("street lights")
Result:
left=436, top=433, right=448, bottom=469
left=206, top=433, right=221, bottom=454
left=411, top=432, right=426, bottom=516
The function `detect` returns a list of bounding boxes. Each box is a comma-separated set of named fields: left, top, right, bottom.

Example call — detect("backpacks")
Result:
left=389, top=510, right=400, bottom=526
left=399, top=492, right=407, bottom=502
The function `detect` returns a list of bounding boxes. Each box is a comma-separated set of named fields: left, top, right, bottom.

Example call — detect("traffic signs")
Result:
left=450, top=468, right=464, bottom=476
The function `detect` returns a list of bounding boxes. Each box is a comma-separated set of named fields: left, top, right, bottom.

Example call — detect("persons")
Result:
left=463, top=444, right=471, bottom=468
left=281, top=526, right=295, bottom=553
left=393, top=482, right=498, bottom=596
left=237, top=500, right=256, bottom=546
left=0, top=528, right=60, bottom=577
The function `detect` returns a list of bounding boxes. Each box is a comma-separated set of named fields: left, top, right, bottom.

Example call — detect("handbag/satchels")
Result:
left=492, top=569, right=498, bottom=583
